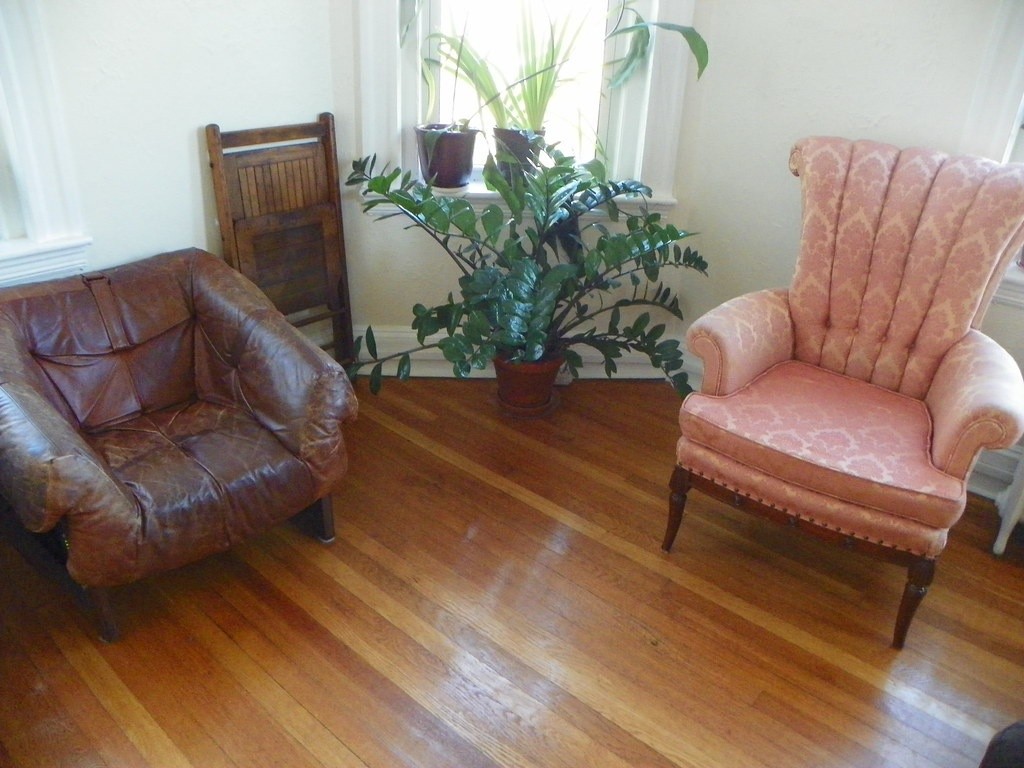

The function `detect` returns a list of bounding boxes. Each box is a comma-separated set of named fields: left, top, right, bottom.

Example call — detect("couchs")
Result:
left=0, top=247, right=359, bottom=643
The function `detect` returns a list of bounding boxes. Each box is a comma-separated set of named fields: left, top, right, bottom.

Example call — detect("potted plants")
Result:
left=334, top=127, right=709, bottom=420
left=421, top=4, right=592, bottom=189
left=413, top=117, right=479, bottom=188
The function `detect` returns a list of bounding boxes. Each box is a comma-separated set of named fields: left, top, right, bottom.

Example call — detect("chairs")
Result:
left=662, top=133, right=1024, bottom=651
left=205, top=112, right=358, bottom=385
